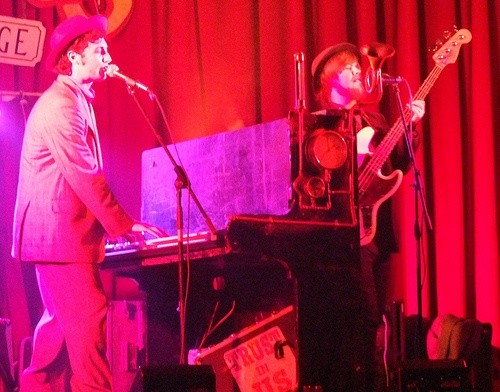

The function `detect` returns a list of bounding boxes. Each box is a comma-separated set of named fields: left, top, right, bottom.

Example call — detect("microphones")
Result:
left=381, top=75, right=403, bottom=84
left=105, top=64, right=148, bottom=90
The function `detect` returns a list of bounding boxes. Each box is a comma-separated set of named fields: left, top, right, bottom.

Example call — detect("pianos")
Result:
left=102, top=107, right=381, bottom=392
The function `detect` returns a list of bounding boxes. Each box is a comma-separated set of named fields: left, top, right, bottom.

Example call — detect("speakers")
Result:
left=227, top=219, right=360, bottom=392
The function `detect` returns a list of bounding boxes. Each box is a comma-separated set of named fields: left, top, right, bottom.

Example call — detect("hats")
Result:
left=49, top=14, right=108, bottom=67
left=311, top=42, right=357, bottom=95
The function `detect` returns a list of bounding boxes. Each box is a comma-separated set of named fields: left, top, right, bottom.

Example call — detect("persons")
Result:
left=12, top=15, right=170, bottom=392
left=311, top=42, right=425, bottom=391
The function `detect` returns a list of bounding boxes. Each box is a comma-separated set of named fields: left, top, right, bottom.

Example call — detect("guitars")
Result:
left=356, top=24, right=475, bottom=248
